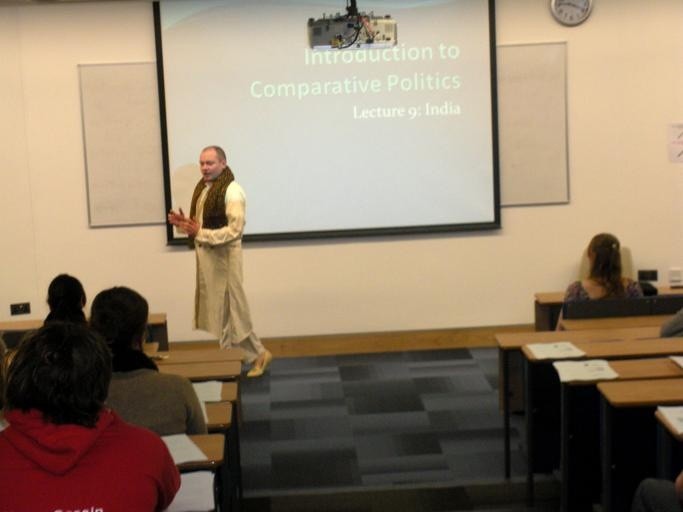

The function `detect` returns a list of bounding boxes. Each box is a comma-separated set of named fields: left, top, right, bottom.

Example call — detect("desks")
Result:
left=1, top=311, right=245, bottom=512
left=493, top=290, right=682, bottom=512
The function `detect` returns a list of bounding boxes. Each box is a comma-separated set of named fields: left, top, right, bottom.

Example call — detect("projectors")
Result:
left=308, top=13, right=398, bottom=49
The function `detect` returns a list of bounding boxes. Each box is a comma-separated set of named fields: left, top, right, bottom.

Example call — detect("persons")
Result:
left=660, top=308, right=683, bottom=338
left=0, top=322, right=180, bottom=512
left=167, top=145, right=273, bottom=378
left=554, top=232, right=644, bottom=331
left=23, top=272, right=87, bottom=346
left=632, top=467, right=683, bottom=512
left=88, top=287, right=208, bottom=437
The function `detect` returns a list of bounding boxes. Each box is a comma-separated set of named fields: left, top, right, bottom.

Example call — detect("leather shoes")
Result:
left=247, top=351, right=273, bottom=377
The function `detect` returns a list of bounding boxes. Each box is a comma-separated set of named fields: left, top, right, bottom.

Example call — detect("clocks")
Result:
left=549, top=1, right=594, bottom=28
